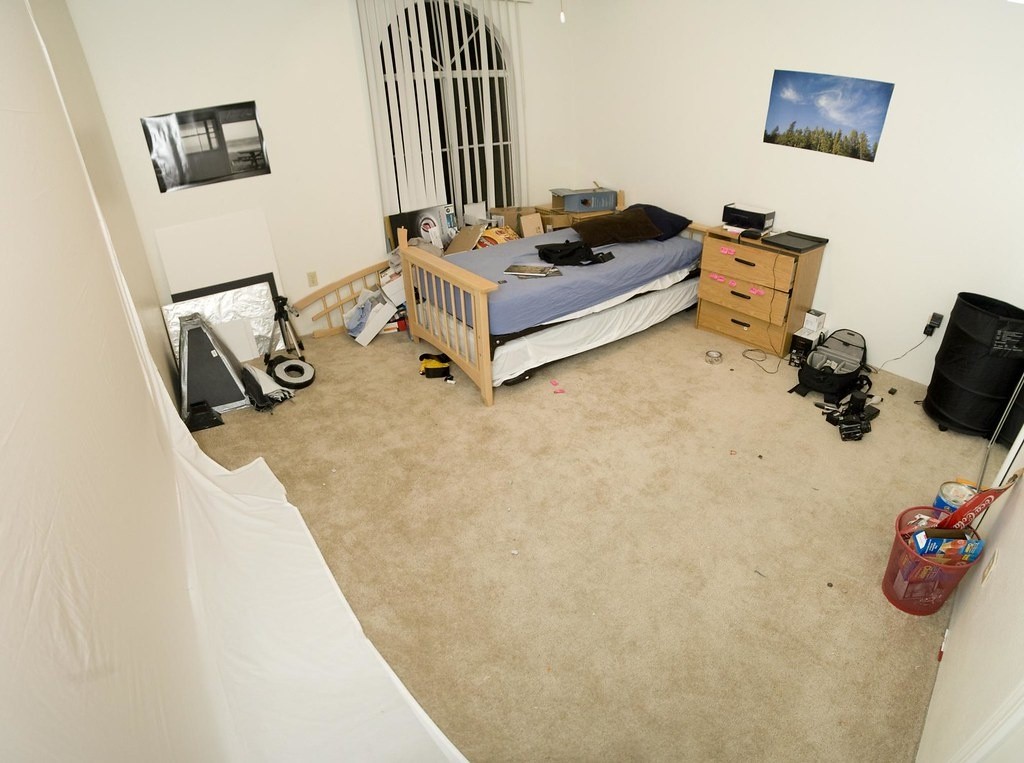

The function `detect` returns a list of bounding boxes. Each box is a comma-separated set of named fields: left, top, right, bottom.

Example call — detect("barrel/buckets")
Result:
left=923, top=291, right=1024, bottom=437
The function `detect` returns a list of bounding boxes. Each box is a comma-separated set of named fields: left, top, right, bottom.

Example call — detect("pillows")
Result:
left=571, top=204, right=693, bottom=248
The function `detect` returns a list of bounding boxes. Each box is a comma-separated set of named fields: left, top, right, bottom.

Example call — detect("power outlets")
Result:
left=923, top=312, right=943, bottom=337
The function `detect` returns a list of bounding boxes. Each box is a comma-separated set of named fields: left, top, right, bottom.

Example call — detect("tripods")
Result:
left=264, top=296, right=305, bottom=366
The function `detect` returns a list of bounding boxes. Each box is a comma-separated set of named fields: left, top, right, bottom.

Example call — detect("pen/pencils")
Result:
left=936, top=628, right=949, bottom=662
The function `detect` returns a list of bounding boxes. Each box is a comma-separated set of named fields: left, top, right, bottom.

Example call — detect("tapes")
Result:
left=705, top=350, right=723, bottom=365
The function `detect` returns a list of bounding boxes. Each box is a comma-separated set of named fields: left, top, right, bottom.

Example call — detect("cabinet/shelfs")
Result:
left=696, top=225, right=830, bottom=359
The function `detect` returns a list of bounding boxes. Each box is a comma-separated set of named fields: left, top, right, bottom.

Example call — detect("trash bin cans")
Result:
left=881, top=505, right=985, bottom=617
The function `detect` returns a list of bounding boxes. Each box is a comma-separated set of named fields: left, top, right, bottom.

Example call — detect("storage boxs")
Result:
left=893, top=528, right=986, bottom=601
left=789, top=310, right=828, bottom=359
left=344, top=186, right=626, bottom=346
left=722, top=203, right=776, bottom=231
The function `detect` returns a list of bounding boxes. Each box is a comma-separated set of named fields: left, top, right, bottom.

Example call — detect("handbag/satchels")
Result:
left=787, top=329, right=872, bottom=406
left=534, top=240, right=615, bottom=266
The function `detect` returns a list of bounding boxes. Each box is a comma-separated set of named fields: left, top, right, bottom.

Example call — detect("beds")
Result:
left=397, top=189, right=707, bottom=406
